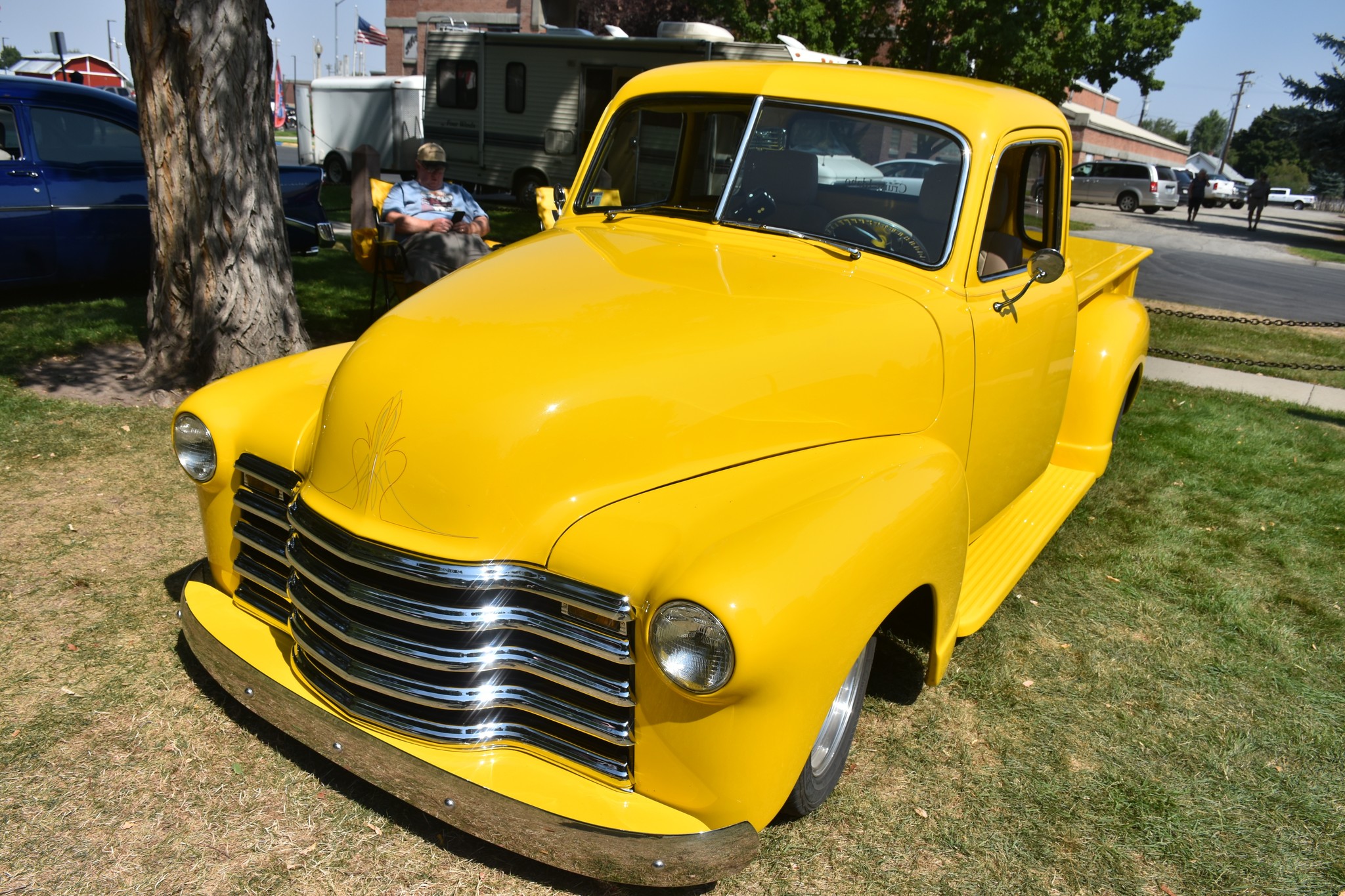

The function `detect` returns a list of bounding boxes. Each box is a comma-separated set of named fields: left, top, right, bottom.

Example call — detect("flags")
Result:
left=357, top=15, right=389, bottom=46
left=274, top=61, right=286, bottom=128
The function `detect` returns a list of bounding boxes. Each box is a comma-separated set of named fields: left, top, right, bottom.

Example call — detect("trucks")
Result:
left=422, top=11, right=864, bottom=218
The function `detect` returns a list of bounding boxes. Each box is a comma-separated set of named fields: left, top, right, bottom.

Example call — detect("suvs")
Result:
left=1031, top=160, right=1180, bottom=215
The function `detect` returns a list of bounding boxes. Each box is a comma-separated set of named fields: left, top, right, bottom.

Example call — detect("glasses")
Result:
left=421, top=162, right=446, bottom=173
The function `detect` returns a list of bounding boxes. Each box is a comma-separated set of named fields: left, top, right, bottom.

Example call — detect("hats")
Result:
left=416, top=143, right=446, bottom=162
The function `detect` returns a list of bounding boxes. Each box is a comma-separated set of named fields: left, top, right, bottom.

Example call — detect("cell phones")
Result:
left=451, top=211, right=465, bottom=223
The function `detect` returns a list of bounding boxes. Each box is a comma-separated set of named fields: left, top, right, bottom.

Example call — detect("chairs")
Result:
left=886, top=163, right=1022, bottom=278
left=742, top=146, right=831, bottom=235
left=351, top=179, right=503, bottom=323
left=534, top=183, right=621, bottom=232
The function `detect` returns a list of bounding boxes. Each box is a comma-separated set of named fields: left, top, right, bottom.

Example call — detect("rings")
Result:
left=461, top=229, right=464, bottom=233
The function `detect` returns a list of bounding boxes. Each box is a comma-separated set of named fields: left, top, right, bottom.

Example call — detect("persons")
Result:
left=381, top=142, right=492, bottom=285
left=1247, top=173, right=1272, bottom=232
left=1187, top=168, right=1210, bottom=223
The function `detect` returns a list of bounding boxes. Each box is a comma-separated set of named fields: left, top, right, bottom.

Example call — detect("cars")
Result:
left=270, top=97, right=297, bottom=131
left=0, top=73, right=335, bottom=304
left=1162, top=168, right=1194, bottom=211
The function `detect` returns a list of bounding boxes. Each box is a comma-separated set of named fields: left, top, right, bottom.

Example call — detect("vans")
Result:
left=91, top=86, right=138, bottom=102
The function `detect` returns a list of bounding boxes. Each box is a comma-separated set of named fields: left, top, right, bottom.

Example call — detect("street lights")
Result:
left=315, top=40, right=323, bottom=78
left=107, top=19, right=123, bottom=62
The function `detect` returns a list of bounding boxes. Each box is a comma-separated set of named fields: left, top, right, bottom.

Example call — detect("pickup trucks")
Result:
left=1266, top=187, right=1317, bottom=211
left=1215, top=179, right=1251, bottom=210
left=170, top=60, right=1156, bottom=884
left=1189, top=171, right=1235, bottom=209
